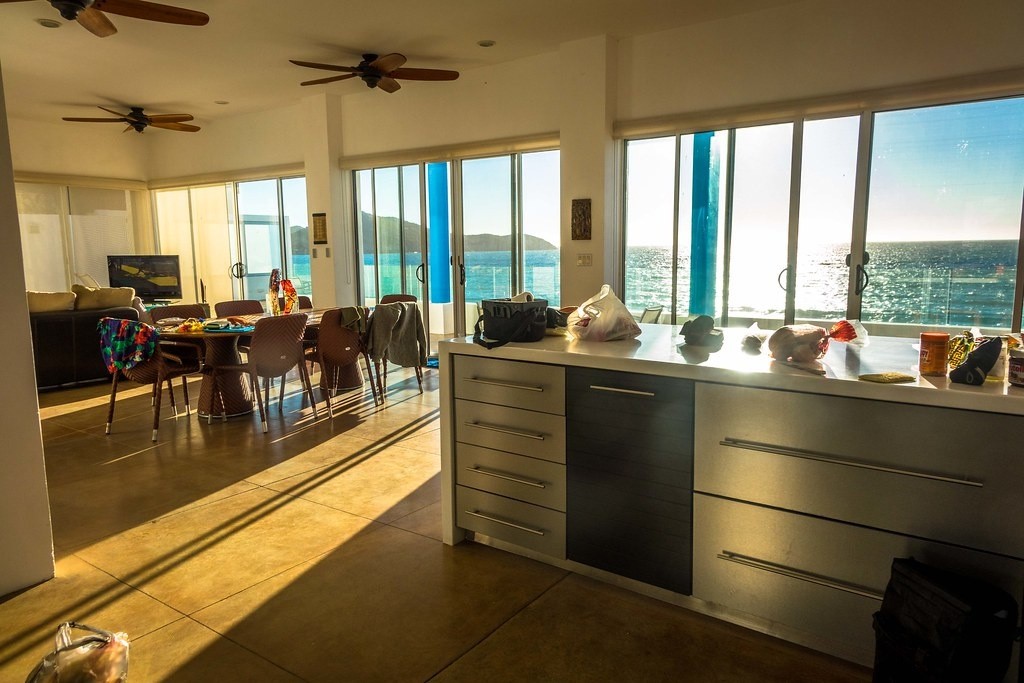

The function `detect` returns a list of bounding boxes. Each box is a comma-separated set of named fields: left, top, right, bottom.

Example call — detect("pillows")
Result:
left=25, top=290, right=77, bottom=313
left=72, top=284, right=135, bottom=311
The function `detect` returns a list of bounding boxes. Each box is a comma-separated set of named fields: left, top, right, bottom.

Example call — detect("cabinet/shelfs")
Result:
left=450, top=352, right=567, bottom=562
left=565, top=365, right=695, bottom=598
left=691, top=380, right=1024, bottom=683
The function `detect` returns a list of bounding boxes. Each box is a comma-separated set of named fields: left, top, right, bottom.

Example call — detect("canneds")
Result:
left=1008, top=350, right=1024, bottom=387
left=920, top=332, right=950, bottom=376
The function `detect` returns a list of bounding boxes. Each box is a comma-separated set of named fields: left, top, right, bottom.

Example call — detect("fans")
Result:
left=288, top=52, right=459, bottom=94
left=61, top=106, right=201, bottom=132
left=1, top=0, right=210, bottom=38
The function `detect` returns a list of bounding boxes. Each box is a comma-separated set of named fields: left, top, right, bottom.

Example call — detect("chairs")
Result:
left=638, top=306, right=663, bottom=325
left=98, top=316, right=227, bottom=443
left=278, top=307, right=378, bottom=418
left=278, top=296, right=317, bottom=375
left=207, top=312, right=318, bottom=433
left=215, top=300, right=306, bottom=402
left=375, top=294, right=423, bottom=388
left=149, top=304, right=209, bottom=415
left=334, top=301, right=424, bottom=404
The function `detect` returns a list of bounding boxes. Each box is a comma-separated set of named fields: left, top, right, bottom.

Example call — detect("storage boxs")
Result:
left=870, top=556, right=1022, bottom=682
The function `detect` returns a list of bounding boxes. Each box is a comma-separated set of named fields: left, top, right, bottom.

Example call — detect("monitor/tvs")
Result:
left=107, top=255, right=183, bottom=304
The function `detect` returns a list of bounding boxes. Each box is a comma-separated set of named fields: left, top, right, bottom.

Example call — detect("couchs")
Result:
left=29, top=306, right=139, bottom=392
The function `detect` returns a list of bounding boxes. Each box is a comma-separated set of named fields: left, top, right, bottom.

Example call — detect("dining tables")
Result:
left=153, top=306, right=374, bottom=419
